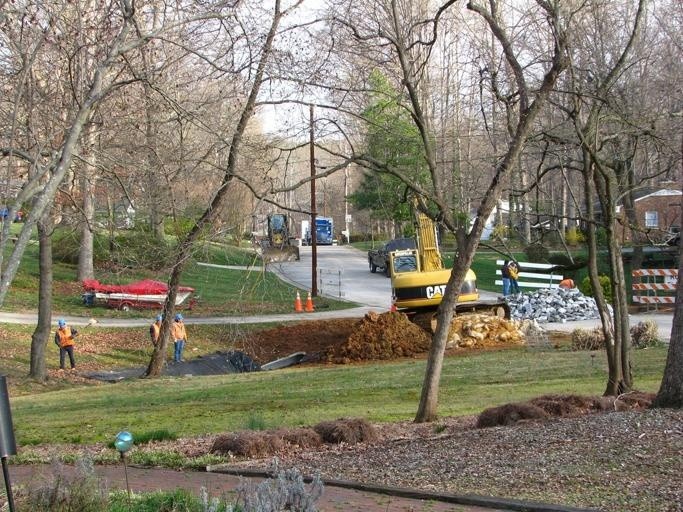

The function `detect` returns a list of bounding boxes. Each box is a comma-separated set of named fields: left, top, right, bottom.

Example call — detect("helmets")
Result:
left=175, top=314, right=184, bottom=320
left=59, top=319, right=65, bottom=326
left=155, top=315, right=162, bottom=321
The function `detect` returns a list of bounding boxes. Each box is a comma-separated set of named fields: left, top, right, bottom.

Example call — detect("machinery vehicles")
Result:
left=80, top=279, right=195, bottom=312
left=387, top=193, right=509, bottom=337
left=257, top=214, right=299, bottom=264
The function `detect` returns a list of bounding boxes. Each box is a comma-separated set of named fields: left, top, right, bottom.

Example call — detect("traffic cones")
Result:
left=294, top=292, right=303, bottom=313
left=304, top=292, right=314, bottom=312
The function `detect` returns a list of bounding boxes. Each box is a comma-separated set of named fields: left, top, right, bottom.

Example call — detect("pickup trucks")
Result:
left=367, top=238, right=418, bottom=278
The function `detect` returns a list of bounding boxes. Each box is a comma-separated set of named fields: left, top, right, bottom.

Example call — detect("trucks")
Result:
left=305, top=218, right=333, bottom=245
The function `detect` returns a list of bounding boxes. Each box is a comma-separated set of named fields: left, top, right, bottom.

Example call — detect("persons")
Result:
left=559, top=279, right=575, bottom=289
left=500, top=260, right=510, bottom=297
left=169, top=312, right=188, bottom=364
left=150, top=314, right=163, bottom=346
left=54, top=318, right=80, bottom=370
left=508, top=263, right=521, bottom=294
